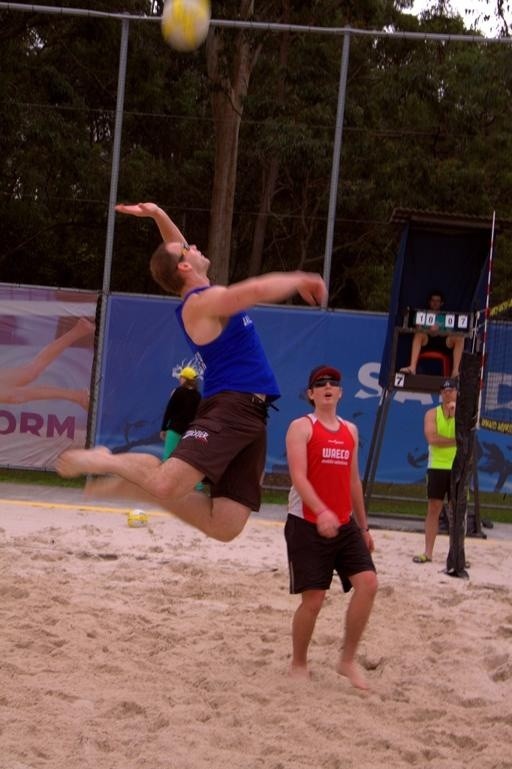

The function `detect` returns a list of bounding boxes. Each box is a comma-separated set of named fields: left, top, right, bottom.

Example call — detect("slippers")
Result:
left=413, top=554, right=431, bottom=563
left=400, top=367, right=413, bottom=374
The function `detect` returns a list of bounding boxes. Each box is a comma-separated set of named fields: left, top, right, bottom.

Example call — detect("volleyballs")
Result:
left=159, top=0, right=210, bottom=51
left=128, top=509, right=147, bottom=528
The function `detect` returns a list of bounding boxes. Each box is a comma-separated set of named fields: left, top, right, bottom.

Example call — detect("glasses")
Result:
left=313, top=378, right=339, bottom=388
left=171, top=243, right=190, bottom=272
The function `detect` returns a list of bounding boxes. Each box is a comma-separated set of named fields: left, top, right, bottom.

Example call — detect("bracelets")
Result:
left=315, top=508, right=329, bottom=516
left=362, top=528, right=369, bottom=533
left=365, top=528, right=370, bottom=532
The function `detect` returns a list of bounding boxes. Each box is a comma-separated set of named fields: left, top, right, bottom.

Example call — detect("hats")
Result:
left=441, top=379, right=456, bottom=390
left=309, top=365, right=340, bottom=389
left=177, top=367, right=198, bottom=380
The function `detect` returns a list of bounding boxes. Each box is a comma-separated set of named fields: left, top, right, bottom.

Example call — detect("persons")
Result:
left=399, top=291, right=465, bottom=377
left=411, top=380, right=472, bottom=563
left=0, top=315, right=97, bottom=412
left=59, top=202, right=326, bottom=542
left=279, top=364, right=378, bottom=691
left=158, top=367, right=202, bottom=491
